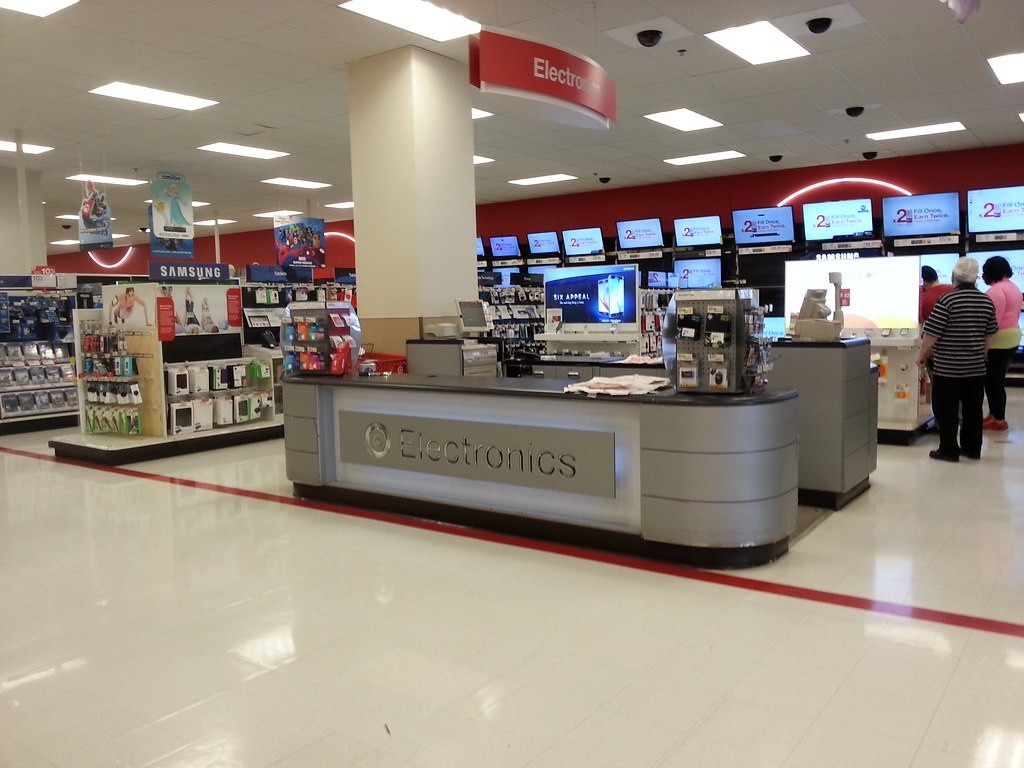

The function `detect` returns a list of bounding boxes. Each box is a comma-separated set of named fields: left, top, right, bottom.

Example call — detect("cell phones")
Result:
left=597, top=274, right=625, bottom=320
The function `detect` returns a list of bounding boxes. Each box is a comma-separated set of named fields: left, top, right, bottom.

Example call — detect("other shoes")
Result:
left=959, top=448, right=980, bottom=460
left=929, top=449, right=959, bottom=462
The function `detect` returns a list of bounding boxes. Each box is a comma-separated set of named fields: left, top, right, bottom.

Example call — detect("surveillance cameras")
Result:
left=140, top=228, right=147, bottom=231
left=637, top=30, right=662, bottom=47
left=599, top=178, right=610, bottom=183
left=769, top=156, right=782, bottom=162
left=862, top=152, right=878, bottom=159
left=845, top=107, right=864, bottom=117
left=807, top=18, right=832, bottom=34
left=62, top=225, right=71, bottom=229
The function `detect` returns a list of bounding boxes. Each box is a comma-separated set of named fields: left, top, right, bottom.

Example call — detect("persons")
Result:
left=163, top=286, right=183, bottom=333
left=921, top=266, right=954, bottom=320
left=201, top=298, right=220, bottom=333
left=184, top=286, right=203, bottom=334
left=915, top=257, right=999, bottom=462
left=980, top=256, right=1024, bottom=430
left=109, top=287, right=152, bottom=326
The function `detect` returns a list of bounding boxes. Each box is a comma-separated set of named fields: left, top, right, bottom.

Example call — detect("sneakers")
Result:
left=982, top=414, right=1009, bottom=433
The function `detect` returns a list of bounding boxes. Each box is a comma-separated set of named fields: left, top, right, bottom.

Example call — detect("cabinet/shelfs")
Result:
left=532, top=365, right=668, bottom=381
left=245, top=344, right=284, bottom=422
left=0, top=340, right=81, bottom=435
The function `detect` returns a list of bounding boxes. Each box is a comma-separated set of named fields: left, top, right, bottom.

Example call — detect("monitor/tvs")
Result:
left=882, top=191, right=962, bottom=238
left=731, top=204, right=795, bottom=244
left=966, top=185, right=1024, bottom=234
left=802, top=198, right=874, bottom=241
left=456, top=300, right=490, bottom=332
left=475, top=214, right=725, bottom=289
left=798, top=288, right=827, bottom=319
